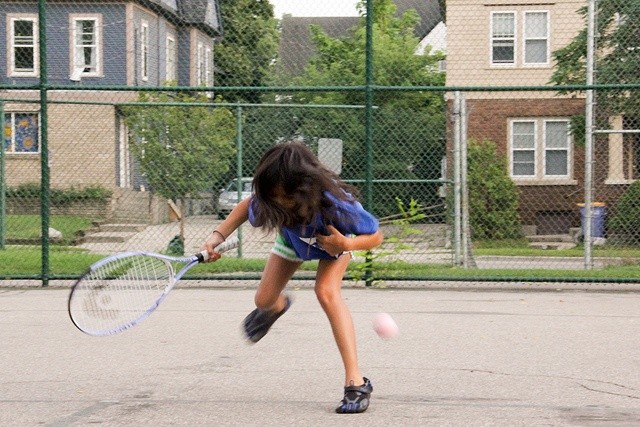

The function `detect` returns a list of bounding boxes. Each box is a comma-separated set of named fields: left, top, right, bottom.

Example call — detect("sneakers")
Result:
left=241, top=295, right=292, bottom=342
left=337, top=377, right=373, bottom=413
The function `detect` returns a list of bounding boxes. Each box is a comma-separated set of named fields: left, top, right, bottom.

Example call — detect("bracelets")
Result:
left=213, top=230, right=225, bottom=241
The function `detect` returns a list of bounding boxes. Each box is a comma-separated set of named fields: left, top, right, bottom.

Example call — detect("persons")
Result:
left=199, top=139, right=383, bottom=414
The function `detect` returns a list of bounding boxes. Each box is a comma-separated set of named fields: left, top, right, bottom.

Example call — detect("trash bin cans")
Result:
left=576, top=201, right=604, bottom=241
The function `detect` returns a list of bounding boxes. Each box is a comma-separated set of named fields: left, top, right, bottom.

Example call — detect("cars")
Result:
left=216, top=177, right=255, bottom=219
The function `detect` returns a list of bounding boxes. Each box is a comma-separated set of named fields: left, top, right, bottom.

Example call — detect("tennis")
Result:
left=65, top=234, right=243, bottom=337
left=376, top=312, right=396, bottom=337
